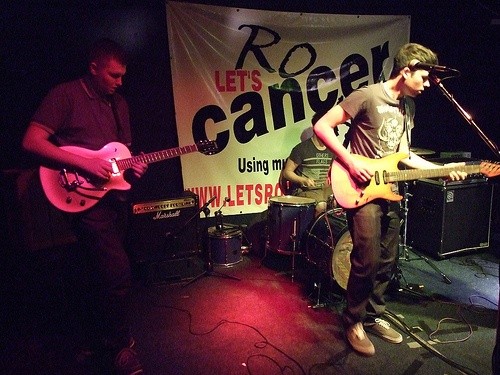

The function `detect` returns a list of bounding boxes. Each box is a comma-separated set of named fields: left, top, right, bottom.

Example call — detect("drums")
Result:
left=208, top=225, right=244, bottom=265
left=265, top=196, right=317, bottom=255
left=306, top=208, right=354, bottom=294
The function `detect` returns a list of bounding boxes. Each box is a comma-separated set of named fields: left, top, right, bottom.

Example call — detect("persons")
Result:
left=24, top=44, right=148, bottom=374
left=283, top=110, right=338, bottom=200
left=316, top=46, right=468, bottom=355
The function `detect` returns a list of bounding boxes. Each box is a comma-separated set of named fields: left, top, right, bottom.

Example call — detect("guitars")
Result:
left=331, top=152, right=500, bottom=210
left=39, top=138, right=217, bottom=213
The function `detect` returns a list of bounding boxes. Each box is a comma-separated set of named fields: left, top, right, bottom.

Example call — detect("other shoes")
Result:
left=114, top=339, right=142, bottom=375
left=363, top=318, right=402, bottom=345
left=74, top=345, right=93, bottom=366
left=345, top=322, right=376, bottom=356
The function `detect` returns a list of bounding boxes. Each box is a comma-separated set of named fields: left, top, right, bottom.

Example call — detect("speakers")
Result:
left=126, top=188, right=200, bottom=286
left=401, top=181, right=492, bottom=258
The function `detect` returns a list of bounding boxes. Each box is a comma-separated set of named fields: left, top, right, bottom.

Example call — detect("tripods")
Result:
left=397, top=178, right=452, bottom=285
left=181, top=195, right=242, bottom=287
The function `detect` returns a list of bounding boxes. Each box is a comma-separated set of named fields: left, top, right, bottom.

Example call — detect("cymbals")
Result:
left=410, top=147, right=435, bottom=155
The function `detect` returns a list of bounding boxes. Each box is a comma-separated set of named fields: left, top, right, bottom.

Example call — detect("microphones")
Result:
left=408, top=58, right=453, bottom=72
